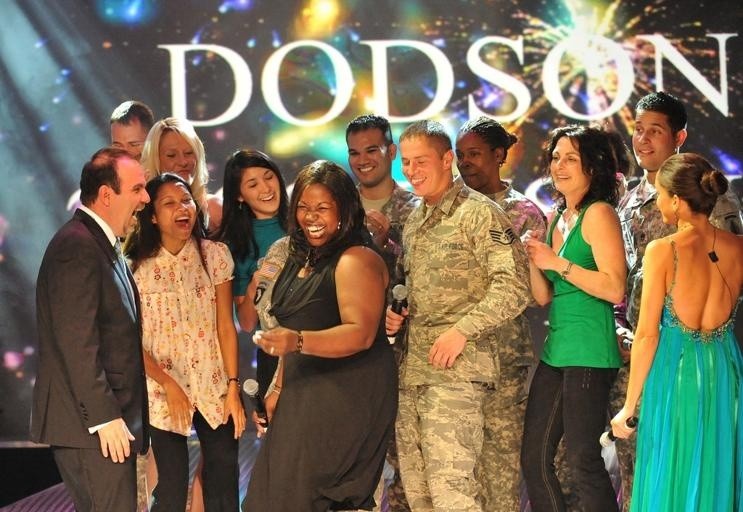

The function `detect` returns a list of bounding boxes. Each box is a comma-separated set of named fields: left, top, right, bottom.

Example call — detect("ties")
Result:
left=114, top=237, right=127, bottom=276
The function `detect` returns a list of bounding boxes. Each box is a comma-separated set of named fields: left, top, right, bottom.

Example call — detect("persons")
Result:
left=67, top=100, right=314, bottom=511
left=609, top=154, right=743, bottom=512
left=341, top=115, right=425, bottom=509
left=385, top=116, right=625, bottom=511
left=29, top=143, right=152, bottom=511
left=237, top=159, right=398, bottom=510
left=598, top=90, right=743, bottom=511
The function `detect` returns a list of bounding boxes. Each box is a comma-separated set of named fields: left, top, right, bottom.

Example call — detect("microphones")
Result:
left=599, top=416, right=639, bottom=448
left=243, top=378, right=269, bottom=428
left=387, top=283, right=409, bottom=344
